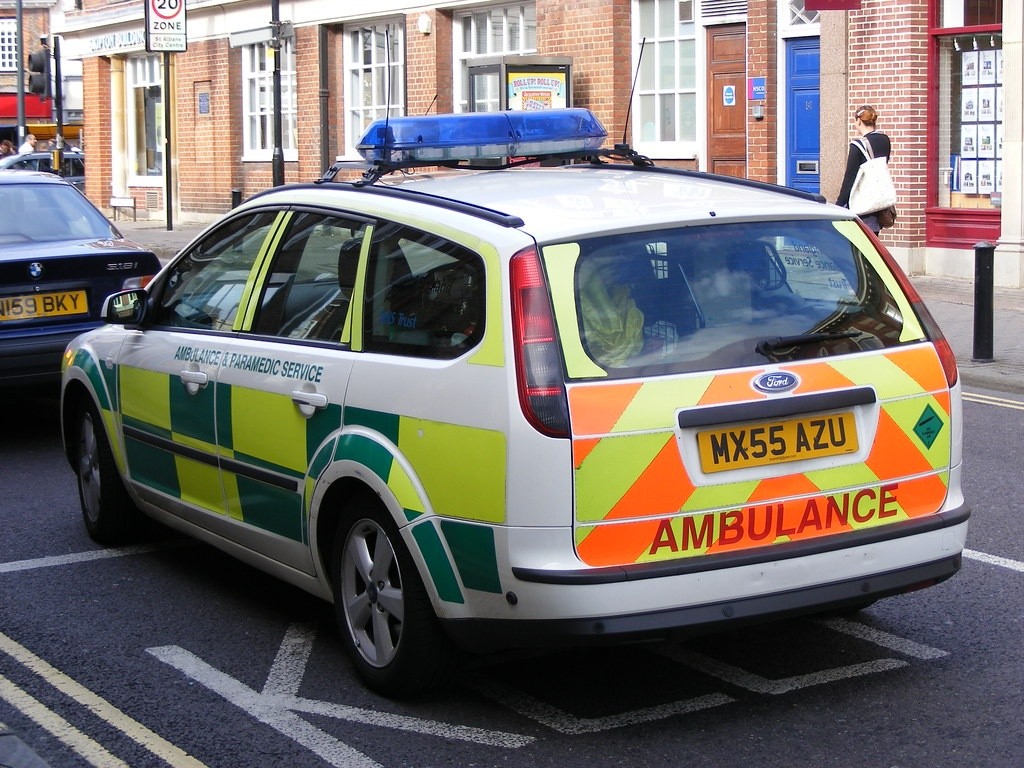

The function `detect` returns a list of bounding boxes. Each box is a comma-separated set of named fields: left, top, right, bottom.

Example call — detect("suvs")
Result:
left=0, top=152, right=85, bottom=192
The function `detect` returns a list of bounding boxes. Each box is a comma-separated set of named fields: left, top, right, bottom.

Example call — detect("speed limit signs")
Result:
left=150, top=0, right=183, bottom=19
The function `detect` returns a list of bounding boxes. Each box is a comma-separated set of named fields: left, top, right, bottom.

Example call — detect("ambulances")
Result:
left=60, top=109, right=970, bottom=705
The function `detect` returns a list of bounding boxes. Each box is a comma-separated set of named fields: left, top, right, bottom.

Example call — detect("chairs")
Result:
left=589, top=245, right=655, bottom=285
left=20, top=207, right=71, bottom=237
left=317, top=237, right=417, bottom=341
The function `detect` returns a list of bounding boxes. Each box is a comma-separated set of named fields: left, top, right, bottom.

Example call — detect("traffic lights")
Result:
left=28, top=49, right=51, bottom=103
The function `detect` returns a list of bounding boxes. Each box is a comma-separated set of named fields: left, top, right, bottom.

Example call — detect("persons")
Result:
left=0, top=134, right=58, bottom=155
left=835, top=105, right=891, bottom=237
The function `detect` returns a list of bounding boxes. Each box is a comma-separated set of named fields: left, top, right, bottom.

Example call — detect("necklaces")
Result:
left=863, top=131, right=875, bottom=137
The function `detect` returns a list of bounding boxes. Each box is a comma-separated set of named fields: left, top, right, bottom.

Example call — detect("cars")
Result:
left=1, top=168, right=165, bottom=425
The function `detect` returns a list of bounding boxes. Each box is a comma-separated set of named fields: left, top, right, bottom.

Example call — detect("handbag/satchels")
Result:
left=873, top=205, right=896, bottom=228
left=848, top=136, right=897, bottom=216
